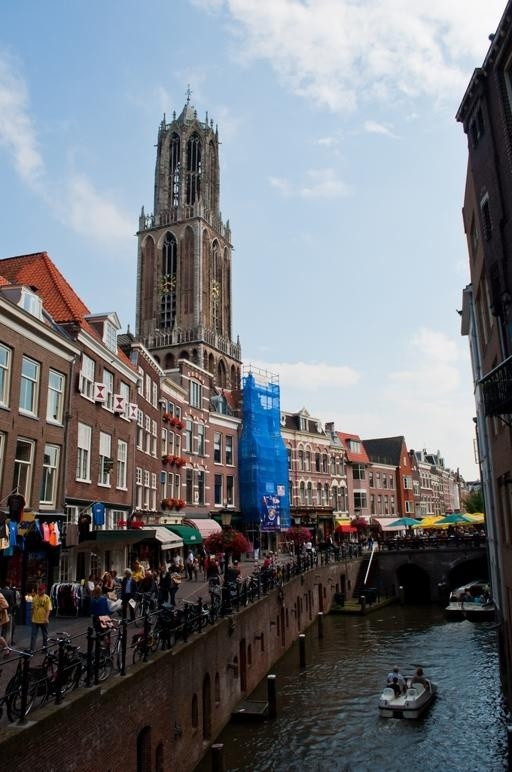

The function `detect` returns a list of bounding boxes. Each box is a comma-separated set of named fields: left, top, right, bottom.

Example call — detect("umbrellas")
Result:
left=381, top=512, right=485, bottom=530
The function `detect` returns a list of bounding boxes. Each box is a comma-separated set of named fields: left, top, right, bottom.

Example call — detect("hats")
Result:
left=38, top=584, right=46, bottom=592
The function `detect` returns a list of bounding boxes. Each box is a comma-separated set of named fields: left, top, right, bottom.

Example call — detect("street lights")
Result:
left=291, top=509, right=305, bottom=571
left=217, top=503, right=238, bottom=618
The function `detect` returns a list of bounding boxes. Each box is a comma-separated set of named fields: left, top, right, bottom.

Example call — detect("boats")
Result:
left=378, top=675, right=434, bottom=724
left=444, top=577, right=497, bottom=623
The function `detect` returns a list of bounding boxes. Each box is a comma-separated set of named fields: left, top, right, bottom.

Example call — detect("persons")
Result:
left=411, top=668, right=428, bottom=688
left=386, top=676, right=401, bottom=697
left=387, top=666, right=406, bottom=685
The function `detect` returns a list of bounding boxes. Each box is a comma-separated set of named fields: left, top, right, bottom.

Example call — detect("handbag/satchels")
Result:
left=171, top=576, right=182, bottom=585
